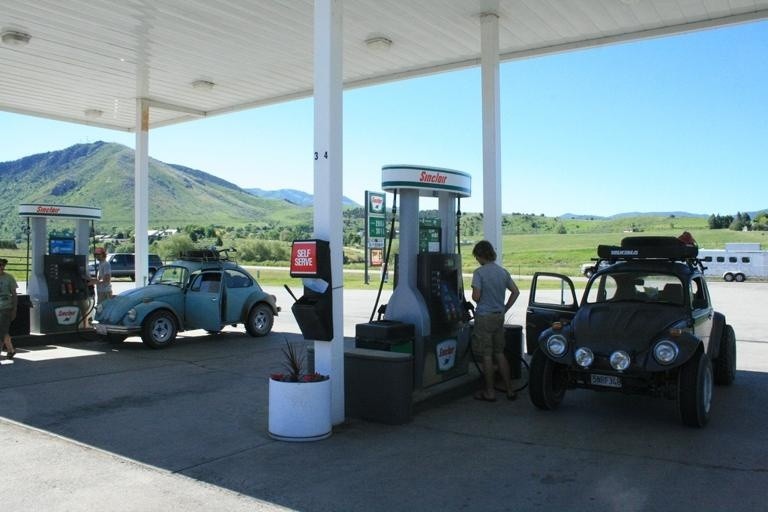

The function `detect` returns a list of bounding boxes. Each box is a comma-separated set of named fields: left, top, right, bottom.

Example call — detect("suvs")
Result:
left=89, top=253, right=162, bottom=283
left=524, top=231, right=737, bottom=429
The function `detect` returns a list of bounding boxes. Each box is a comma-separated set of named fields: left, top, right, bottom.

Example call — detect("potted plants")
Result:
left=269, top=337, right=332, bottom=442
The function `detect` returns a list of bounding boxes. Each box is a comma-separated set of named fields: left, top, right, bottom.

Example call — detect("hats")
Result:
left=94, top=248, right=105, bottom=254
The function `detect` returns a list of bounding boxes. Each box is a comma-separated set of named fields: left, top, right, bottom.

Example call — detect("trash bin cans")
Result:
left=9, top=294, right=30, bottom=336
left=356, top=320, right=416, bottom=393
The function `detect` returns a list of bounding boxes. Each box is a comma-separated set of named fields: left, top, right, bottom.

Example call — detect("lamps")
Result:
left=366, top=37, right=392, bottom=49
left=191, top=80, right=215, bottom=91
left=84, top=109, right=103, bottom=118
left=0, top=29, right=33, bottom=48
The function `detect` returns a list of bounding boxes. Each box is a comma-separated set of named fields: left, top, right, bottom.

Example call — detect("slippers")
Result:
left=5, top=350, right=17, bottom=359
left=473, top=391, right=518, bottom=402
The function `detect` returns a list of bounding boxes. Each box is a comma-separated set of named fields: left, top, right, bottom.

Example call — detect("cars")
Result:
left=89, top=259, right=280, bottom=349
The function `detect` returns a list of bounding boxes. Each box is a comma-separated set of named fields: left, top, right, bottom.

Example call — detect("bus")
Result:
left=696, top=248, right=767, bottom=282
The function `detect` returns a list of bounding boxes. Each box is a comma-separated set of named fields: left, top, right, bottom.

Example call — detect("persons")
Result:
left=472, top=239, right=519, bottom=403
left=78, top=245, right=113, bottom=306
left=1, top=257, right=20, bottom=360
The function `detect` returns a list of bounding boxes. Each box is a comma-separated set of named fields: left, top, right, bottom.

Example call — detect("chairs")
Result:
left=663, top=283, right=683, bottom=292
